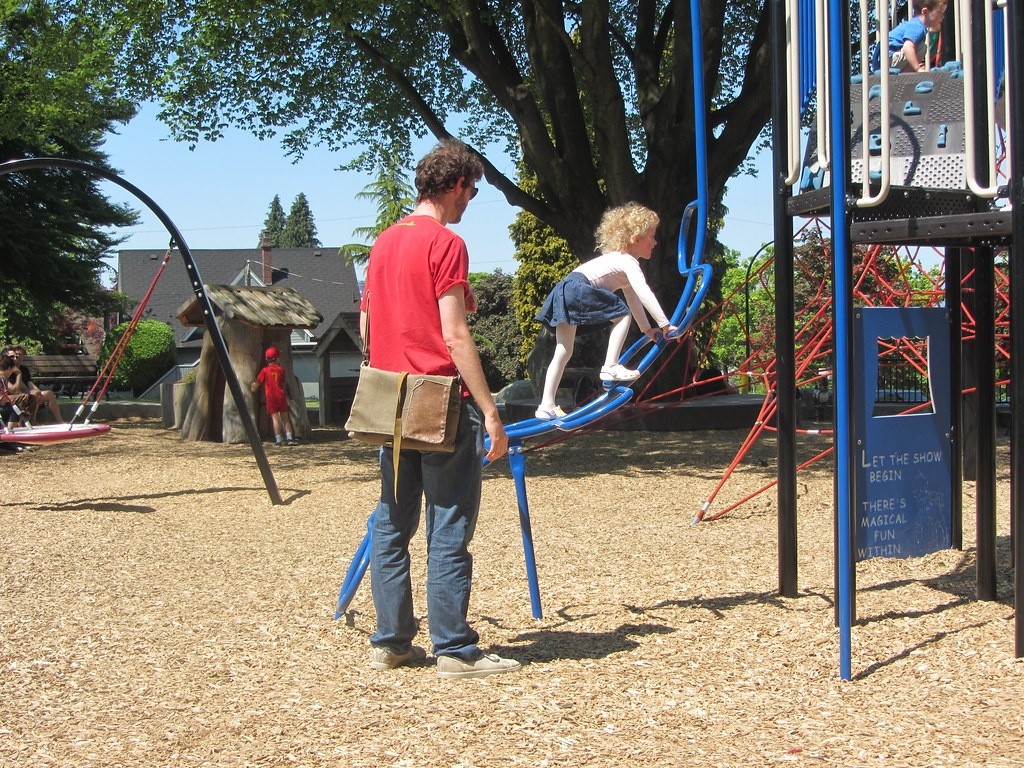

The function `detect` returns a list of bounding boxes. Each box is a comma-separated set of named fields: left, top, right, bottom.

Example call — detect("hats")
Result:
left=266, top=346, right=284, bottom=362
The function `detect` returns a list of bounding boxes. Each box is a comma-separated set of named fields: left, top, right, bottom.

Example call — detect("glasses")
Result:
left=10, top=355, right=19, bottom=359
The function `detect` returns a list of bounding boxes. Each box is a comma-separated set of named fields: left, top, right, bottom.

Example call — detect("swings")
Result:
left=0, top=244, right=174, bottom=445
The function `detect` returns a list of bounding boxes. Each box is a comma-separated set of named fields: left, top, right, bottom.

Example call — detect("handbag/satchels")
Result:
left=344, top=360, right=462, bottom=452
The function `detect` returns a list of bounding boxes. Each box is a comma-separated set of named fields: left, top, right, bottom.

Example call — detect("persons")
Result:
left=251, top=347, right=293, bottom=446
left=689, top=348, right=724, bottom=396
left=14, top=346, right=65, bottom=425
left=0, top=358, right=33, bottom=435
left=873, top=0, right=947, bottom=75
left=533, top=204, right=680, bottom=422
left=1, top=348, right=39, bottom=427
left=360, top=140, right=521, bottom=678
left=796, top=387, right=801, bottom=399
left=812, top=378, right=829, bottom=407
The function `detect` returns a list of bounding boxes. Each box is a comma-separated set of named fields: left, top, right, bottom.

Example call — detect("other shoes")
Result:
left=273, top=442, right=282, bottom=447
left=287, top=439, right=298, bottom=445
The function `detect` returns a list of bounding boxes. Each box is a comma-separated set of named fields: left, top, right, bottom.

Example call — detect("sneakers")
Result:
left=599, top=364, right=641, bottom=381
left=373, top=646, right=426, bottom=668
left=437, top=651, right=522, bottom=678
left=536, top=405, right=582, bottom=434
